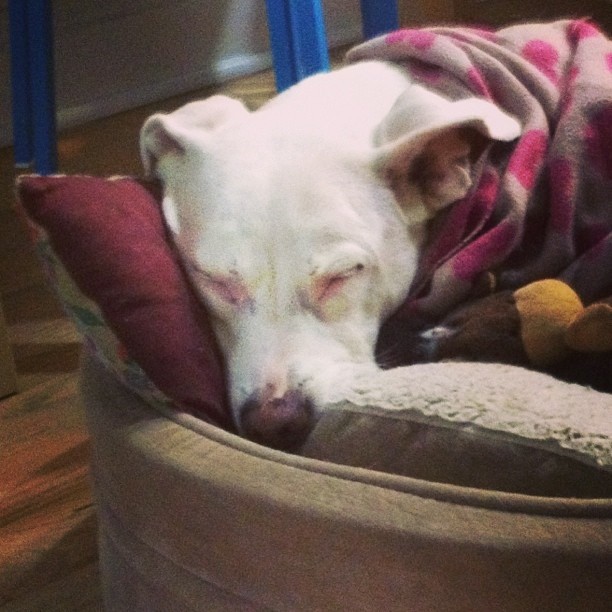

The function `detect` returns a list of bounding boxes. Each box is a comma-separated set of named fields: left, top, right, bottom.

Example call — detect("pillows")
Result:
left=7, top=174, right=241, bottom=436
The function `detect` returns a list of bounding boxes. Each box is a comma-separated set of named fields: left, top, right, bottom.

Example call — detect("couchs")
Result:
left=77, top=346, right=612, bottom=611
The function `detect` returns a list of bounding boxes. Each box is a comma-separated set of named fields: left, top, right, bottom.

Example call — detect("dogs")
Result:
left=139, top=57, right=521, bottom=452
